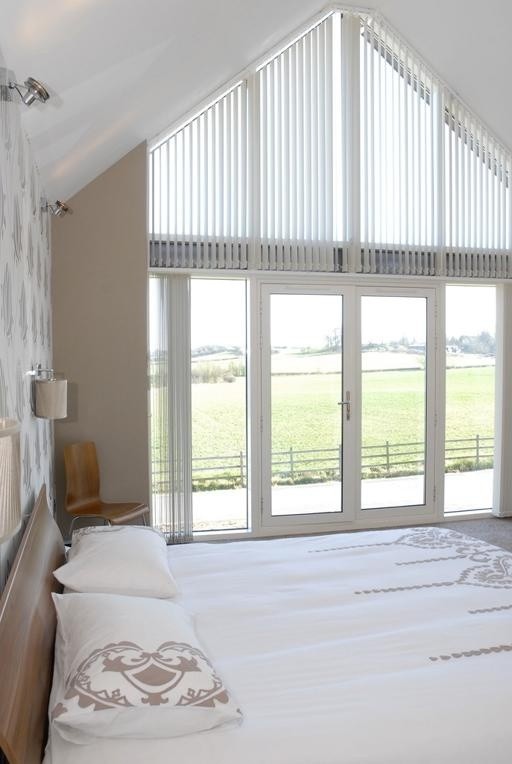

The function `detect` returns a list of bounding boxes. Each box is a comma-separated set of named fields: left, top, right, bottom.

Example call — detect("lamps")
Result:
left=35, top=364, right=68, bottom=420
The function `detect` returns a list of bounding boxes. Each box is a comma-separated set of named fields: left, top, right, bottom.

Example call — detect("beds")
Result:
left=0, top=483, right=512, bottom=764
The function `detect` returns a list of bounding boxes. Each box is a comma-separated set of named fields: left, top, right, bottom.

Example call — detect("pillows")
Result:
left=49, top=590, right=243, bottom=745
left=51, top=524, right=177, bottom=598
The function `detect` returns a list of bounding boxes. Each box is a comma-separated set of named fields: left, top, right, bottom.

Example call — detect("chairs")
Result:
left=62, top=441, right=150, bottom=546
